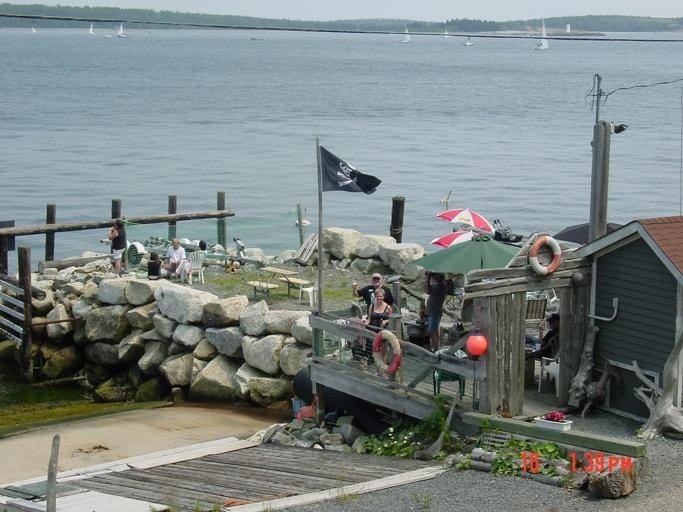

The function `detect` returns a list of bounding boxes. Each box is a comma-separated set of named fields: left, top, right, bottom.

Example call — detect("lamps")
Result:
left=467, top=307, right=488, bottom=355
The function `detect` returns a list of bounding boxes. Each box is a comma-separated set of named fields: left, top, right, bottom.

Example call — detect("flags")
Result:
left=319, top=144, right=382, bottom=195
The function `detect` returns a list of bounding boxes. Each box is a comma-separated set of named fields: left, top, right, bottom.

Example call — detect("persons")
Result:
left=524, top=313, right=560, bottom=390
left=351, top=272, right=395, bottom=320
left=364, top=288, right=392, bottom=365
left=165, top=238, right=186, bottom=272
left=170, top=240, right=205, bottom=283
left=147, top=251, right=161, bottom=280
left=108, top=219, right=126, bottom=277
left=423, top=270, right=446, bottom=353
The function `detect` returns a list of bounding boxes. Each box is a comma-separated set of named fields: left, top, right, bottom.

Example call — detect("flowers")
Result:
left=541, top=409, right=565, bottom=422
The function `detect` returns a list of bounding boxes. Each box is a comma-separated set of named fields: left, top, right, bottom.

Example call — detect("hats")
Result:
left=371, top=273, right=380, bottom=278
left=545, top=314, right=560, bottom=322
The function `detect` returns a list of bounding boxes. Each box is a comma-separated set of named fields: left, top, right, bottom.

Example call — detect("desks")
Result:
left=260, top=267, right=299, bottom=285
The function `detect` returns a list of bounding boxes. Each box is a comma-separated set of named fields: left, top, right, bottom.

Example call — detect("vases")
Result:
left=533, top=417, right=573, bottom=431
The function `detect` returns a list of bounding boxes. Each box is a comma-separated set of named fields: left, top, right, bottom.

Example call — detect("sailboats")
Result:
left=400, top=26, right=412, bottom=43
left=116, top=23, right=130, bottom=38
left=531, top=18, right=549, bottom=50
left=88, top=22, right=97, bottom=35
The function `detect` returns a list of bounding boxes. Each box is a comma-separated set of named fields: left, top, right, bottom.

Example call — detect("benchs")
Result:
left=278, top=277, right=310, bottom=299
left=247, top=281, right=279, bottom=305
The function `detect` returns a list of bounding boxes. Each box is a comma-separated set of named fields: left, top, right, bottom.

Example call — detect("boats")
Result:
left=463, top=37, right=475, bottom=46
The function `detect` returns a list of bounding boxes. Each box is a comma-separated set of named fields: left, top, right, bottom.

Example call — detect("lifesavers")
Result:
left=528, top=236, right=562, bottom=274
left=372, top=330, right=402, bottom=374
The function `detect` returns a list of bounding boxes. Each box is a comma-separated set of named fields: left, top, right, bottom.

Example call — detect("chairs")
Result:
left=299, top=279, right=319, bottom=307
left=180, top=251, right=207, bottom=286
left=538, top=354, right=560, bottom=398
left=432, top=349, right=469, bottom=396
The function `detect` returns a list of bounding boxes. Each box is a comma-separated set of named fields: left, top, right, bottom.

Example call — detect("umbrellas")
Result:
left=436, top=208, right=496, bottom=236
left=429, top=230, right=478, bottom=248
left=408, top=231, right=524, bottom=276
